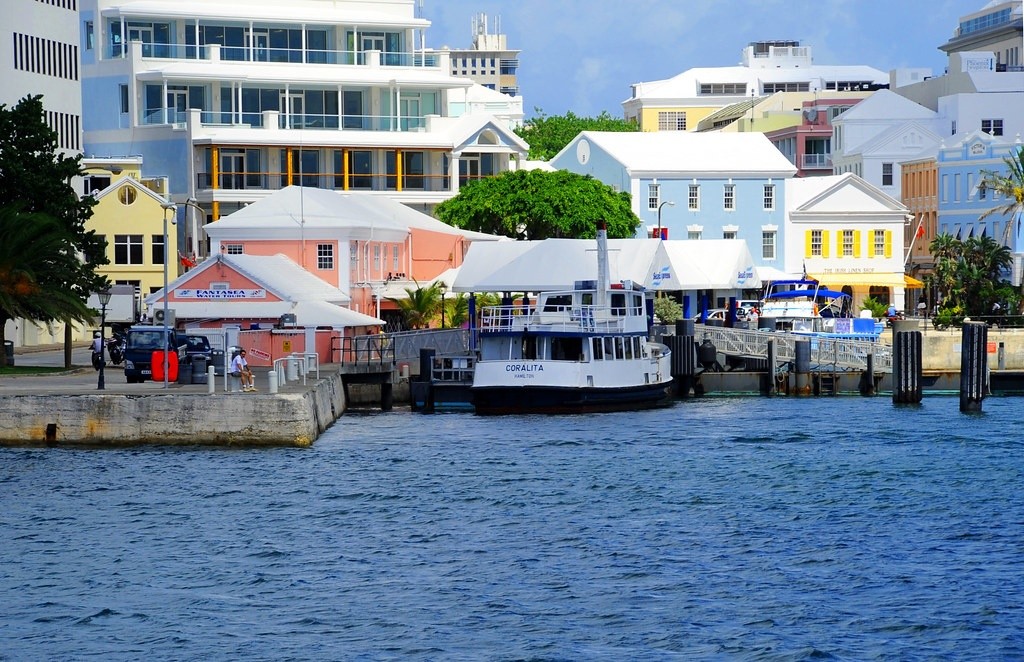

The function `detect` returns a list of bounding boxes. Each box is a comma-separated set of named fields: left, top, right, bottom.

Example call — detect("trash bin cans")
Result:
left=192, top=354, right=208, bottom=383
left=178, top=364, right=192, bottom=383
left=4, top=340, right=14, bottom=366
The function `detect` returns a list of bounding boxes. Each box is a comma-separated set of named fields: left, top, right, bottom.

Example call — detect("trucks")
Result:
left=124, top=326, right=227, bottom=385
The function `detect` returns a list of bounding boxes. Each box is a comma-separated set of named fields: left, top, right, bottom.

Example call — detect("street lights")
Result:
left=156, top=201, right=180, bottom=389
left=181, top=195, right=198, bottom=275
left=95, top=284, right=113, bottom=390
left=654, top=197, right=676, bottom=240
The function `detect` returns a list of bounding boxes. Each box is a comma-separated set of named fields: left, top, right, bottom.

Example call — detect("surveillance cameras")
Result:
left=171, top=219, right=176, bottom=225
left=906, top=214, right=915, bottom=220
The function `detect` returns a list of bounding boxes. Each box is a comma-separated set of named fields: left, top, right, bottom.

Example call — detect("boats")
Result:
left=470, top=220, right=675, bottom=413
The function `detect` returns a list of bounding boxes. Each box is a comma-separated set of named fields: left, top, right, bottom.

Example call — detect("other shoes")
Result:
left=243, top=388, right=250, bottom=391
left=249, top=387, right=256, bottom=391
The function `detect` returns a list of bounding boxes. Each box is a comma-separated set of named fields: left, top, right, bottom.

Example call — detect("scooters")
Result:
left=883, top=308, right=905, bottom=327
left=89, top=348, right=105, bottom=370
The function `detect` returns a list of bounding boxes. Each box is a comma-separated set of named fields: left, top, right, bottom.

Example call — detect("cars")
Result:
left=690, top=299, right=857, bottom=324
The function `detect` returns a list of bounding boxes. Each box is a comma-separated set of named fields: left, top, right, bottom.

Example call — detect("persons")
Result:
left=746, top=305, right=758, bottom=320
left=88, top=332, right=106, bottom=367
left=963, top=314, right=971, bottom=322
left=888, top=303, right=901, bottom=323
left=717, top=313, right=723, bottom=319
left=230, top=350, right=256, bottom=392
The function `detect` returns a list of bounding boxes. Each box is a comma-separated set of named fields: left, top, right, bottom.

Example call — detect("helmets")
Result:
left=890, top=303, right=894, bottom=306
left=95, top=332, right=102, bottom=337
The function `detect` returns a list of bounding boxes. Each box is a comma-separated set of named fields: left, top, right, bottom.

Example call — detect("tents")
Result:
left=763, top=280, right=852, bottom=301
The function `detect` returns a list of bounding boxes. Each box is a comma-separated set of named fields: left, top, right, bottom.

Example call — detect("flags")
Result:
left=915, top=225, right=926, bottom=240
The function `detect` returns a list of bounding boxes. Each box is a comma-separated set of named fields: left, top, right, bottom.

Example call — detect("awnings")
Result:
left=756, top=267, right=808, bottom=284
left=805, top=272, right=924, bottom=289
